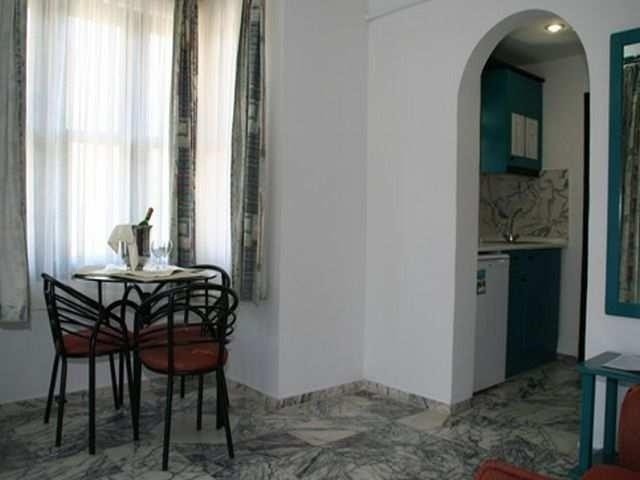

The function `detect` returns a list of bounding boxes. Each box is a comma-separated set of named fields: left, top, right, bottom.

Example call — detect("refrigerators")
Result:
left=474, top=256, right=510, bottom=392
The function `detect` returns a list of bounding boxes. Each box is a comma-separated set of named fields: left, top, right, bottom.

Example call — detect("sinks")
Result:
left=481, top=238, right=552, bottom=244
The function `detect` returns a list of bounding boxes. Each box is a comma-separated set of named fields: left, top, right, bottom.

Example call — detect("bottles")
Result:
left=140, top=207, right=153, bottom=225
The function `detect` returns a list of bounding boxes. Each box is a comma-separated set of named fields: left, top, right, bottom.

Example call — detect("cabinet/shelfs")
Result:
left=481, top=66, right=545, bottom=178
left=501, top=247, right=561, bottom=379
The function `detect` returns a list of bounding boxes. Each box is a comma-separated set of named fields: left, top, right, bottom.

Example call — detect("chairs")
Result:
left=475, top=384, right=640, bottom=480
left=133, top=283, right=239, bottom=471
left=41, top=272, right=138, bottom=446
left=152, top=265, right=231, bottom=431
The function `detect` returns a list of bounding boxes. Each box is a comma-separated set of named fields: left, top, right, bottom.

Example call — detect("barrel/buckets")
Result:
left=120, top=226, right=151, bottom=265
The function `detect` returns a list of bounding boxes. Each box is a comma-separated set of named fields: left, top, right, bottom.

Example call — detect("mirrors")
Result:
left=605, top=28, right=640, bottom=319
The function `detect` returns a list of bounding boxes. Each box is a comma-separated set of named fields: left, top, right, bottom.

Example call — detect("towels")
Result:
left=108, top=224, right=138, bottom=271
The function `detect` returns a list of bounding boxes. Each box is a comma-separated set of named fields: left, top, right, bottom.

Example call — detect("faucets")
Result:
left=502, top=205, right=523, bottom=243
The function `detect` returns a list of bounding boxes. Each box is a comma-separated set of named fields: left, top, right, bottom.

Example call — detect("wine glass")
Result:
left=151, top=239, right=173, bottom=273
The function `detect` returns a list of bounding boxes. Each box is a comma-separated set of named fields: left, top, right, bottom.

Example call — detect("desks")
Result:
left=568, top=351, right=640, bottom=480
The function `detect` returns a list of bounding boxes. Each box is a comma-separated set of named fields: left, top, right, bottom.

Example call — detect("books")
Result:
left=601, top=352, right=640, bottom=374
